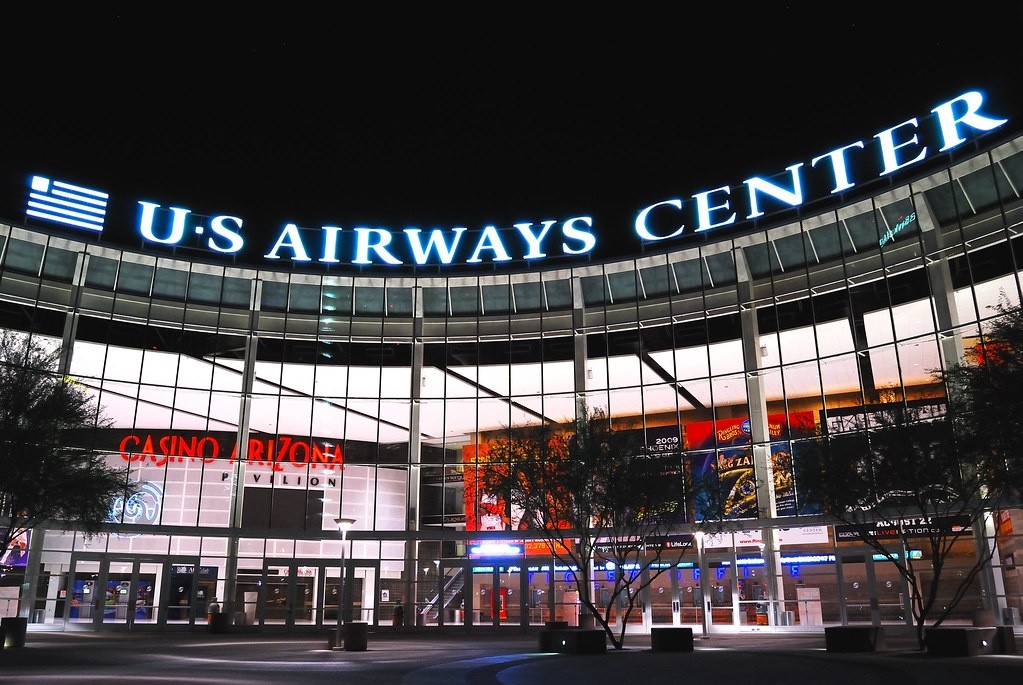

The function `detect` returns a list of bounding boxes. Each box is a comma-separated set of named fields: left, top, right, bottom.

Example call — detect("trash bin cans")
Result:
left=344, top=622, right=368, bottom=651
left=208, top=603, right=220, bottom=624
left=392, top=606, right=404, bottom=626
left=755, top=602, right=768, bottom=625
left=1, top=618, right=28, bottom=648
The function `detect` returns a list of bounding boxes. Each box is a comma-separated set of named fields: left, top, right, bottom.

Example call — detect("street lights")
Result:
left=333, top=518, right=356, bottom=650
